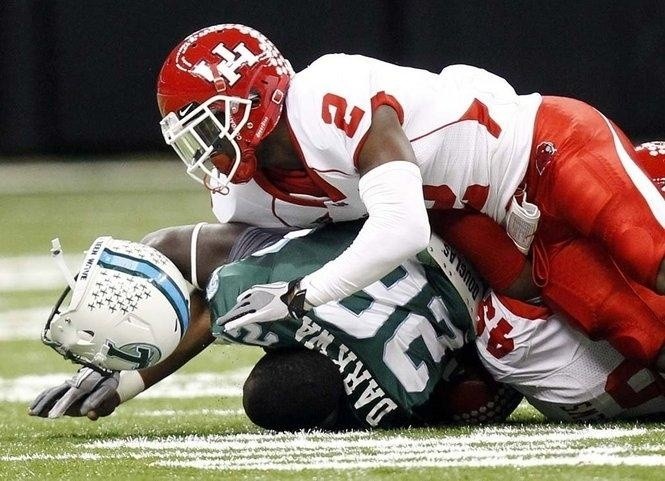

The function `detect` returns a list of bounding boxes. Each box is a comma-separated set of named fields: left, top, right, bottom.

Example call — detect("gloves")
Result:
left=217, top=279, right=304, bottom=332
left=29, top=367, right=120, bottom=421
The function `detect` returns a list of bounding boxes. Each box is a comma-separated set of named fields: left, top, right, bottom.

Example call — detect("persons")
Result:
left=155, top=22, right=665, bottom=381
left=27, top=221, right=525, bottom=431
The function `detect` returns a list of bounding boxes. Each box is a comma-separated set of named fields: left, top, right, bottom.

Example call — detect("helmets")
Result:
left=636, top=138, right=665, bottom=193
left=42, top=237, right=191, bottom=376
left=439, top=377, right=526, bottom=425
left=156, top=24, right=295, bottom=196
